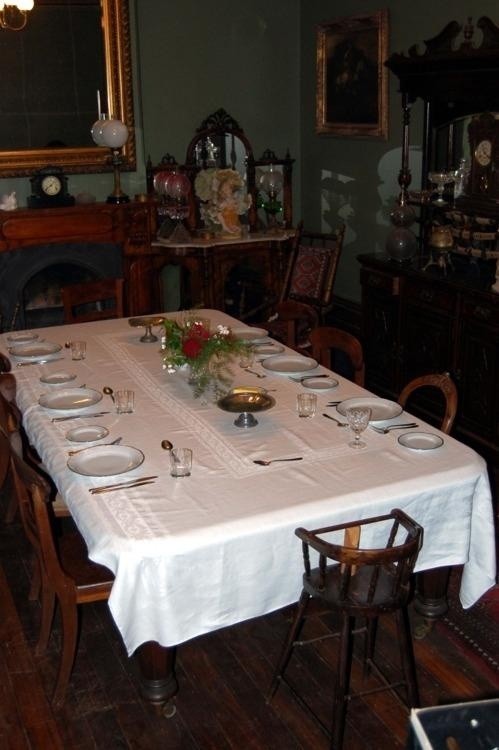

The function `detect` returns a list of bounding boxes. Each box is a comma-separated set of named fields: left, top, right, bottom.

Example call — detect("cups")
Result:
left=297, top=393, right=317, bottom=419
left=71, top=341, right=87, bottom=361
left=114, top=390, right=134, bottom=414
left=170, top=449, right=192, bottom=477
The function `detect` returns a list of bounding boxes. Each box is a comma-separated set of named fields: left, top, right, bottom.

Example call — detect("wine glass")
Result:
left=346, top=407, right=372, bottom=449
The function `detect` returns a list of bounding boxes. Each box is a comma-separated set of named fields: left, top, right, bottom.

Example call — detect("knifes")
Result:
left=55, top=415, right=104, bottom=422
left=92, top=481, right=155, bottom=495
left=53, top=411, right=111, bottom=422
left=89, top=476, right=158, bottom=491
left=17, top=358, right=64, bottom=367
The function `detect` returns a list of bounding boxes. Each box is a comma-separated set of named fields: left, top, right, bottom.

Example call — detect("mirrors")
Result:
left=184, top=106, right=256, bottom=233
left=314, top=8, right=390, bottom=139
left=147, top=152, right=192, bottom=237
left=255, top=147, right=295, bottom=230
left=1, top=0, right=138, bottom=178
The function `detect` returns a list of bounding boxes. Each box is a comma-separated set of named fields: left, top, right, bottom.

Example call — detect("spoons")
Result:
left=322, top=414, right=349, bottom=426
left=65, top=341, right=73, bottom=349
left=162, top=440, right=190, bottom=476
left=105, top=387, right=116, bottom=401
left=253, top=458, right=303, bottom=465
left=244, top=368, right=266, bottom=379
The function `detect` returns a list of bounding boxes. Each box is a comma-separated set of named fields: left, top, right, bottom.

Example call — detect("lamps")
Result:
left=153, top=169, right=178, bottom=238
left=1, top=0, right=35, bottom=31
left=420, top=171, right=456, bottom=276
left=256, top=168, right=266, bottom=231
left=259, top=163, right=285, bottom=233
left=91, top=120, right=107, bottom=147
left=103, top=114, right=129, bottom=205
left=456, top=155, right=470, bottom=195
left=164, top=165, right=192, bottom=244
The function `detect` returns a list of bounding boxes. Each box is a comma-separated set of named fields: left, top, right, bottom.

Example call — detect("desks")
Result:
left=151, top=227, right=299, bottom=325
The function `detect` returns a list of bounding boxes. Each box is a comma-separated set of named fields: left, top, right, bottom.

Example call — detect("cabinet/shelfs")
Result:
left=0, top=195, right=162, bottom=317
left=357, top=261, right=401, bottom=403
left=402, top=268, right=499, bottom=464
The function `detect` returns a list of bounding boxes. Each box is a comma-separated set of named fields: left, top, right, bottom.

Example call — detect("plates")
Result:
left=398, top=432, right=444, bottom=450
left=261, top=356, right=319, bottom=372
left=8, top=342, right=63, bottom=360
left=6, top=333, right=39, bottom=342
left=40, top=370, right=76, bottom=384
left=231, top=327, right=269, bottom=340
left=255, top=345, right=285, bottom=354
left=336, top=397, right=403, bottom=421
left=38, top=387, right=103, bottom=410
left=67, top=444, right=145, bottom=476
left=301, top=377, right=339, bottom=390
left=65, top=425, right=109, bottom=442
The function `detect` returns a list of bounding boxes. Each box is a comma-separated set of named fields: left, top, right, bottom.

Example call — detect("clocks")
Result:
left=26, top=164, right=76, bottom=208
left=467, top=112, right=499, bottom=199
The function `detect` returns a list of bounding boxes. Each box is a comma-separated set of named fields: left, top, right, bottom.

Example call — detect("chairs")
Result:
left=238, top=219, right=347, bottom=350
left=0, top=350, right=36, bottom=489
left=265, top=509, right=424, bottom=750
left=58, top=277, right=127, bottom=327
left=301, top=325, right=365, bottom=390
left=264, top=298, right=320, bottom=349
left=398, top=376, right=458, bottom=434
left=6, top=431, right=116, bottom=715
left=0, top=373, right=72, bottom=601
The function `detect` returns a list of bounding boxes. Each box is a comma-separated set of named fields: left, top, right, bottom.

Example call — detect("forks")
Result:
left=369, top=425, right=419, bottom=434
left=371, top=423, right=416, bottom=430
left=288, top=375, right=328, bottom=382
left=69, top=436, right=123, bottom=456
